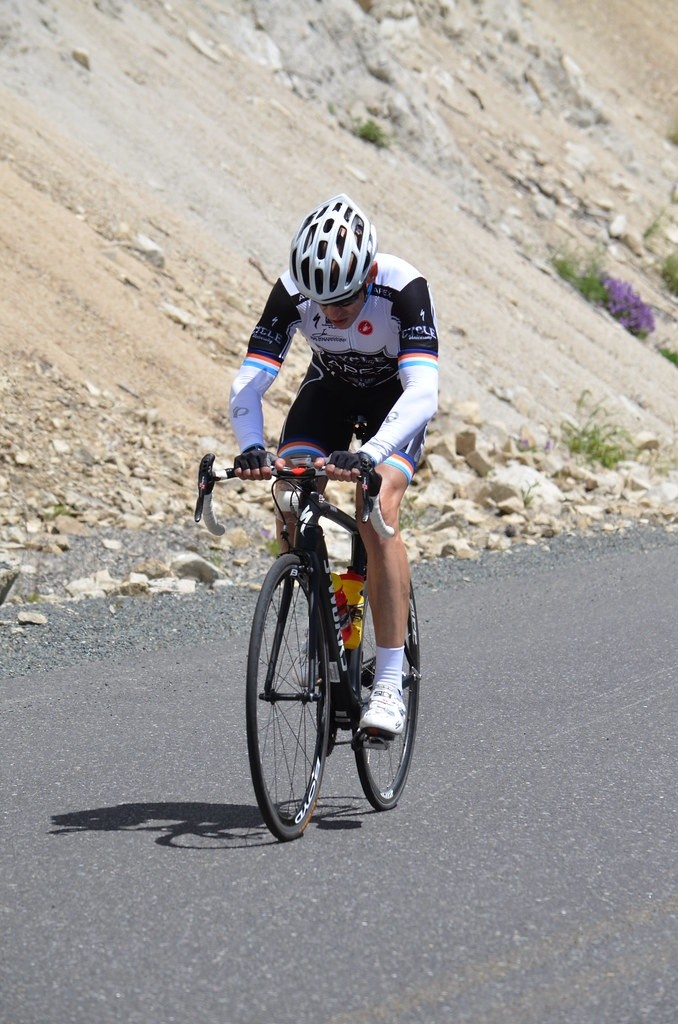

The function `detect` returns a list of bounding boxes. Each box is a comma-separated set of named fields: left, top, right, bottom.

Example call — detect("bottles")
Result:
left=339, top=566, right=363, bottom=650
left=330, top=572, right=353, bottom=641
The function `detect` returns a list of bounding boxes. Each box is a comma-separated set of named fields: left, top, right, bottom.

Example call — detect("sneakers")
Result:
left=292, top=631, right=320, bottom=687
left=359, top=684, right=407, bottom=736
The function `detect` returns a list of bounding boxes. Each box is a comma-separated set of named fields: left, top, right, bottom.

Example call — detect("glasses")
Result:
left=322, top=292, right=360, bottom=308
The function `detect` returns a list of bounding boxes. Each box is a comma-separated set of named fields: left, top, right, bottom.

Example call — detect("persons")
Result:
left=229, top=194, right=439, bottom=738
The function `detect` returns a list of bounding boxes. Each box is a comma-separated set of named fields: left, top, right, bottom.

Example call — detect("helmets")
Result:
left=289, top=193, right=378, bottom=305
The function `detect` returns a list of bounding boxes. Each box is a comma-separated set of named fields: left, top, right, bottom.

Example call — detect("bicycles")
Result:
left=194, top=452, right=422, bottom=843
